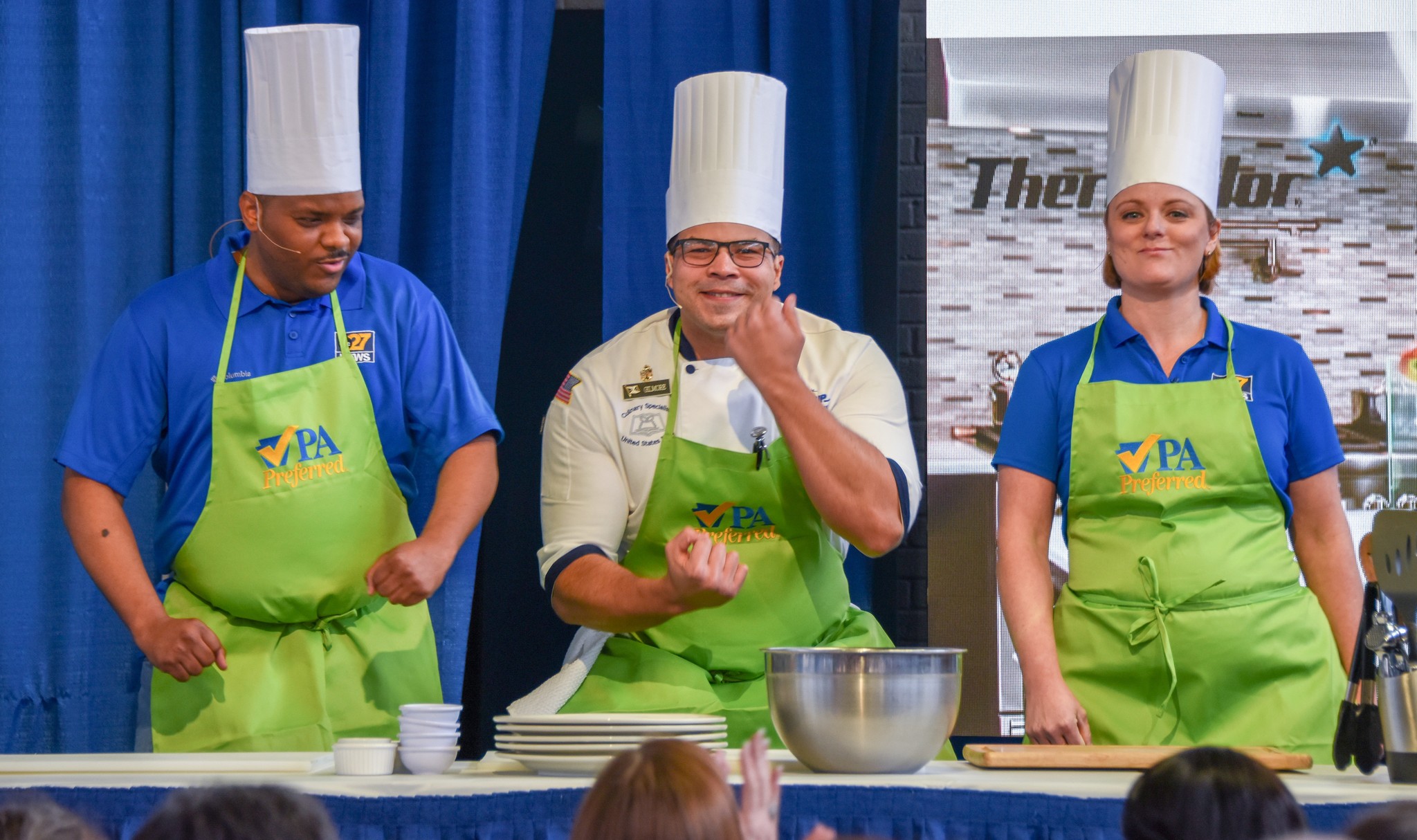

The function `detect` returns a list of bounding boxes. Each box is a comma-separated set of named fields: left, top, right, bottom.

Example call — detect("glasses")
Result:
left=670, top=237, right=779, bottom=268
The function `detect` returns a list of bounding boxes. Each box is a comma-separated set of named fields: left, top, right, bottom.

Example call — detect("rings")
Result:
left=767, top=809, right=776, bottom=820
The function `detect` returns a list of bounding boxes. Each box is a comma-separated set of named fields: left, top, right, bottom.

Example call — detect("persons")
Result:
left=0, top=784, right=339, bottom=840
left=54, top=23, right=502, bottom=751
left=1120, top=746, right=1304, bottom=840
left=570, top=729, right=784, bottom=840
left=509, top=70, right=960, bottom=762
left=988, top=51, right=1367, bottom=767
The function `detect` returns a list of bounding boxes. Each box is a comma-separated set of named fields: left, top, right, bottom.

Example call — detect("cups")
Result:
left=1374, top=664, right=1417, bottom=786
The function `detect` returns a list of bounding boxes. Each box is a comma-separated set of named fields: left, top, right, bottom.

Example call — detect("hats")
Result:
left=246, top=23, right=361, bottom=196
left=666, top=72, right=786, bottom=243
left=1105, top=48, right=1224, bottom=220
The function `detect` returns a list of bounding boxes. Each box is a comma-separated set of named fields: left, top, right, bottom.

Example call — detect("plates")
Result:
left=493, top=711, right=728, bottom=778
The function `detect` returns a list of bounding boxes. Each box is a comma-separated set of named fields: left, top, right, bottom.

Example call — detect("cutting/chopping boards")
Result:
left=961, top=743, right=1313, bottom=771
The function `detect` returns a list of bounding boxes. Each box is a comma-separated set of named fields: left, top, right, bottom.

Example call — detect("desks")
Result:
left=1, top=753, right=1417, bottom=840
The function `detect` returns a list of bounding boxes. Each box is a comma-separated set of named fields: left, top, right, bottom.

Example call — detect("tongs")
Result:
left=1333, top=581, right=1385, bottom=776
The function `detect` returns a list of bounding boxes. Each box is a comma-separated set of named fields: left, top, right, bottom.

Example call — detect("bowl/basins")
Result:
left=759, top=646, right=968, bottom=776
left=332, top=735, right=398, bottom=775
left=396, top=704, right=463, bottom=775
